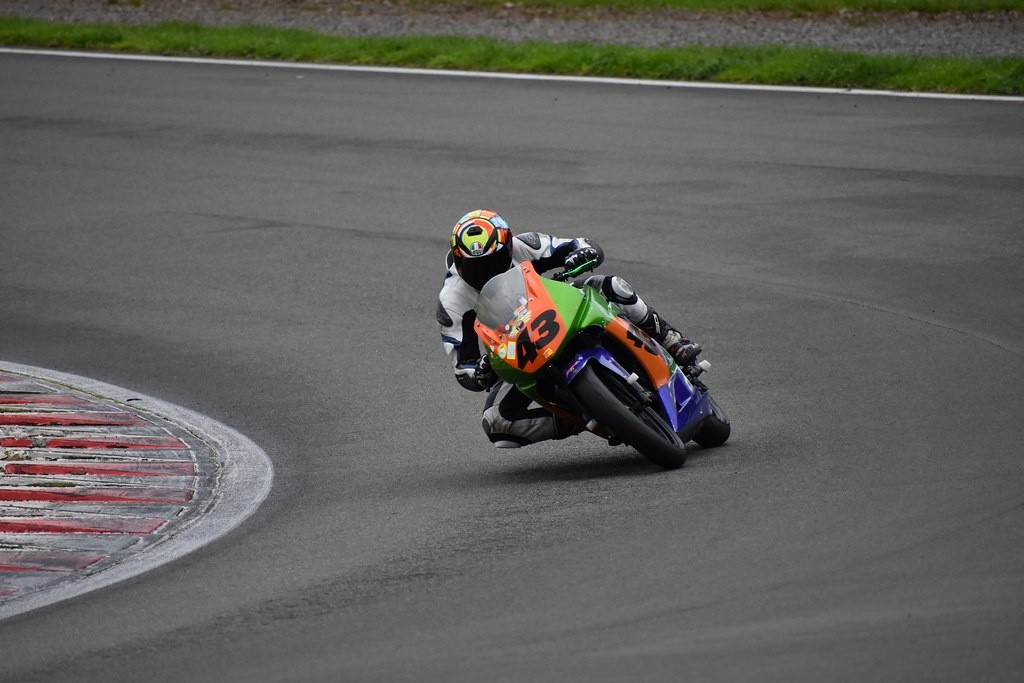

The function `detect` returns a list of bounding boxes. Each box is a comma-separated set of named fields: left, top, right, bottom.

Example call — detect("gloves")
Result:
left=474, top=354, right=498, bottom=385
left=563, top=247, right=598, bottom=274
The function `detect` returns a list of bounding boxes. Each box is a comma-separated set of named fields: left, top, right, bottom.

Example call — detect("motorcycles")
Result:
left=475, top=244, right=732, bottom=469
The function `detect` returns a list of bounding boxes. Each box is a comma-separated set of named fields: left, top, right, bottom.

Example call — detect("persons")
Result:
left=436, top=210, right=702, bottom=447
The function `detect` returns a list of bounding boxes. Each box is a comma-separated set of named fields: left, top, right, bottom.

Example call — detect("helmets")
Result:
left=449, top=209, right=514, bottom=291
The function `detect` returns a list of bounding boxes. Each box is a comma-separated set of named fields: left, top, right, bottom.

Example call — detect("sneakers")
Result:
left=664, top=331, right=703, bottom=367
